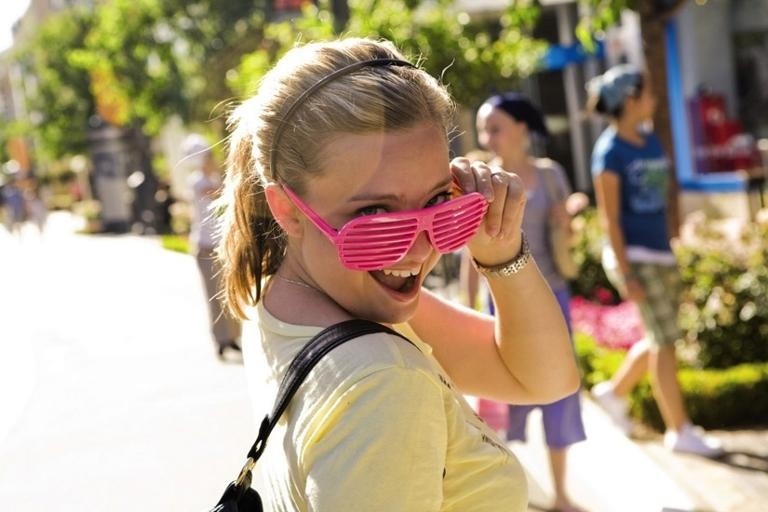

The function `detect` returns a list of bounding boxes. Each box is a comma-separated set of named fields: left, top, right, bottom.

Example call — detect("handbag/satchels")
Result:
left=209, top=320, right=447, bottom=511
left=536, top=158, right=577, bottom=281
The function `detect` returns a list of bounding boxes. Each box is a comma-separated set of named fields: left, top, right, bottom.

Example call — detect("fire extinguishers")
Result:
left=690, top=80, right=726, bottom=174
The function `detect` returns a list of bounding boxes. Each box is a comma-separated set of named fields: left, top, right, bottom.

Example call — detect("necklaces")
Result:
left=269, top=275, right=318, bottom=292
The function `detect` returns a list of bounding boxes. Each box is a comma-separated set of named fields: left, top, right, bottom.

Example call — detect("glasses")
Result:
left=273, top=168, right=490, bottom=272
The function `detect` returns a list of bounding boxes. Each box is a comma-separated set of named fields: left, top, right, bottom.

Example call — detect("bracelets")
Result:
left=467, top=230, right=531, bottom=279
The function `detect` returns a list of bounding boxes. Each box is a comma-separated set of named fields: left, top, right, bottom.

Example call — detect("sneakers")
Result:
left=590, top=379, right=635, bottom=439
left=663, top=420, right=725, bottom=459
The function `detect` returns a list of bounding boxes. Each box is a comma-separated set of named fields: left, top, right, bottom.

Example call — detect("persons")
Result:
left=188, top=130, right=244, bottom=365
left=213, top=34, right=581, bottom=511
left=459, top=91, right=587, bottom=510
left=587, top=56, right=728, bottom=457
